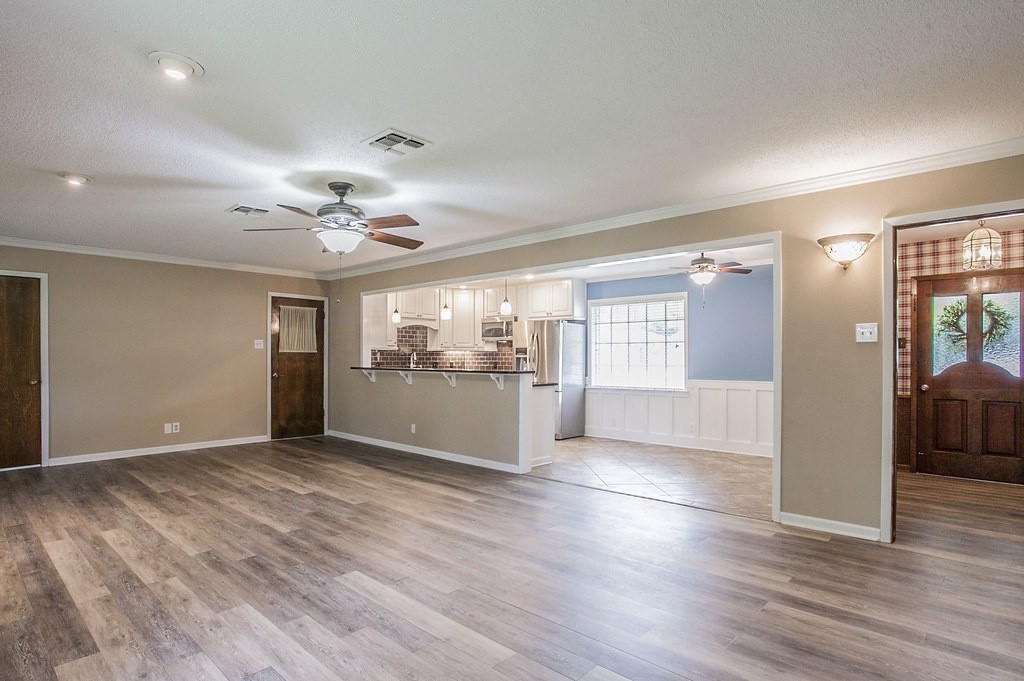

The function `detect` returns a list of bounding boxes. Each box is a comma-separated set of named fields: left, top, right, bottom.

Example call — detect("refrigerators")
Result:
left=534, top=321, right=586, bottom=438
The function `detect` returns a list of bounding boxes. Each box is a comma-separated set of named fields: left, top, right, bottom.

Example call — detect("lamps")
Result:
left=316, top=231, right=365, bottom=304
left=817, top=234, right=875, bottom=270
left=963, top=221, right=1002, bottom=274
left=441, top=285, right=452, bottom=321
left=690, top=272, right=716, bottom=309
left=500, top=277, right=512, bottom=316
left=392, top=291, right=401, bottom=324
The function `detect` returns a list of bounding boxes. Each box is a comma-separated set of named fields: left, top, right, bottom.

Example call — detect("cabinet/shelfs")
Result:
left=454, top=289, right=475, bottom=348
left=439, top=287, right=453, bottom=347
left=397, top=287, right=438, bottom=320
left=483, top=286, right=517, bottom=315
left=474, top=290, right=483, bottom=348
left=526, top=278, right=574, bottom=318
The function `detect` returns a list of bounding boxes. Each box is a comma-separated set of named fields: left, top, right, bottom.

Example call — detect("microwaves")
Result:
left=482, top=319, right=512, bottom=341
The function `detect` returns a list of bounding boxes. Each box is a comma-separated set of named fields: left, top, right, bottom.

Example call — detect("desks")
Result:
left=529, top=382, right=559, bottom=469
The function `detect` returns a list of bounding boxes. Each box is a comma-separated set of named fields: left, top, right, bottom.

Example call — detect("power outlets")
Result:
left=173, top=423, right=180, bottom=433
left=164, top=423, right=172, bottom=434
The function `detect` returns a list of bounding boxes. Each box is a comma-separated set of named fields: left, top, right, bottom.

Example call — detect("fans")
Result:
left=669, top=253, right=752, bottom=275
left=242, top=182, right=423, bottom=250
